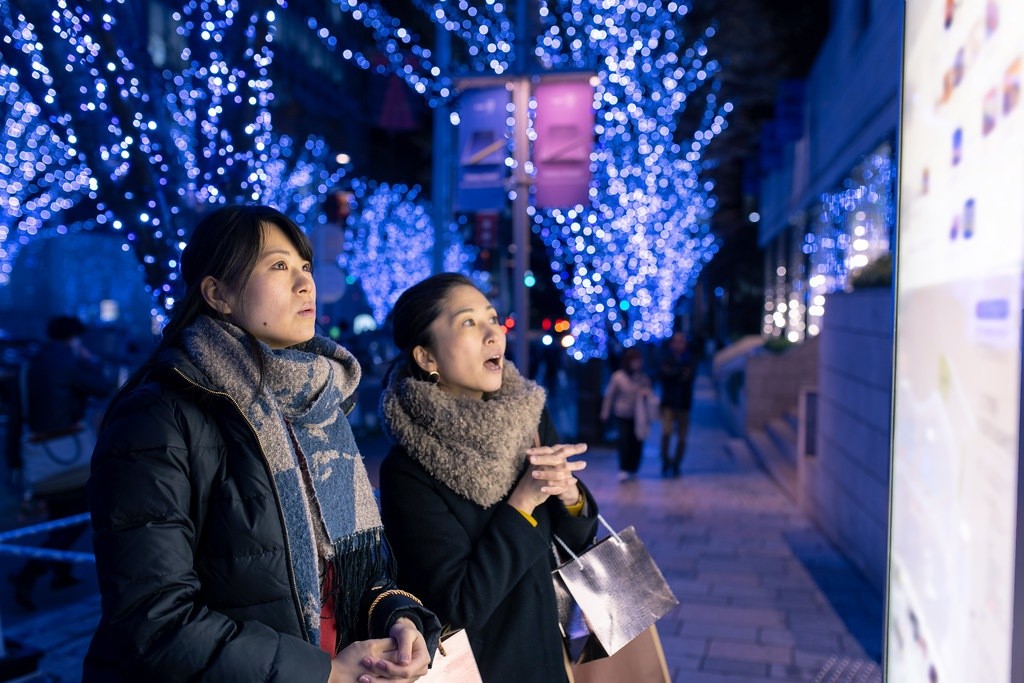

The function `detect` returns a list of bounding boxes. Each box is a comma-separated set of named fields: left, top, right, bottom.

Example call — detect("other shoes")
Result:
left=13, top=573, right=36, bottom=611
left=673, top=463, right=682, bottom=477
left=50, top=571, right=80, bottom=590
left=663, top=463, right=671, bottom=481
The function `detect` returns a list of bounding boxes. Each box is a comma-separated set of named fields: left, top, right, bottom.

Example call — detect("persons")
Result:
left=601, top=351, right=653, bottom=481
left=378, top=272, right=598, bottom=683
left=536, top=346, right=576, bottom=441
left=657, top=332, right=697, bottom=477
left=82, top=204, right=430, bottom=683
left=7, top=318, right=113, bottom=612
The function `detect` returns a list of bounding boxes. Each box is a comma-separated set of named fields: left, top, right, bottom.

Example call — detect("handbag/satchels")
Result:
left=550, top=512, right=679, bottom=657
left=562, top=624, right=671, bottom=683
left=369, top=591, right=484, bottom=683
left=24, top=423, right=96, bottom=500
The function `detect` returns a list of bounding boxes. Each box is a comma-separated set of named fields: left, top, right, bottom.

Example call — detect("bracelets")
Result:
left=579, top=495, right=582, bottom=502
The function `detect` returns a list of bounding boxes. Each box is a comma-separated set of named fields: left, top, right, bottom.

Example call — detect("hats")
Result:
left=48, top=316, right=85, bottom=340
